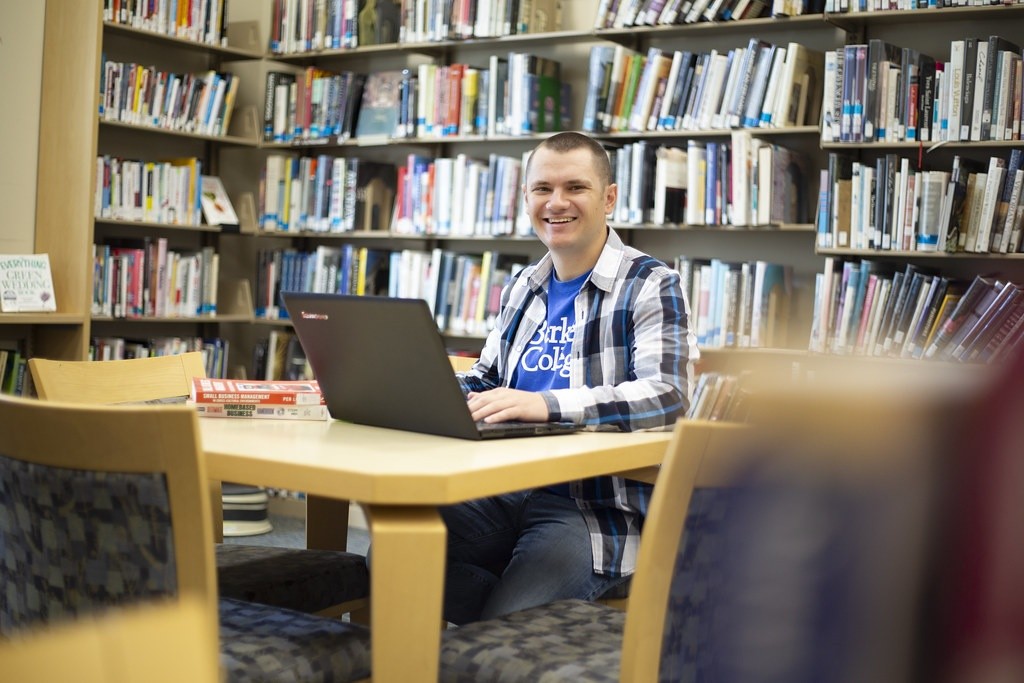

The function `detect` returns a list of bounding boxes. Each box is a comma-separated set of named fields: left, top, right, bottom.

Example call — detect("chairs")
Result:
left=697, top=346, right=983, bottom=440
left=441, top=413, right=928, bottom=683
left=0, top=394, right=372, bottom=683
left=31, top=350, right=370, bottom=627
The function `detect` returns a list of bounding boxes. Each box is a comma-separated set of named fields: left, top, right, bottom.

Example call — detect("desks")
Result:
left=193, top=413, right=675, bottom=683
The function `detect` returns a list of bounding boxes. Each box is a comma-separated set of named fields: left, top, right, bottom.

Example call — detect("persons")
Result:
left=365, top=131, right=699, bottom=620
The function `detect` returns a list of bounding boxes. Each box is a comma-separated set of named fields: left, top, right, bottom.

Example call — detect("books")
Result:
left=0, top=0, right=1024, bottom=540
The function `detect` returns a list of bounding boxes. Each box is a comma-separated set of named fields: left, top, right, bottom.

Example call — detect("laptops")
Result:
left=278, top=289, right=586, bottom=440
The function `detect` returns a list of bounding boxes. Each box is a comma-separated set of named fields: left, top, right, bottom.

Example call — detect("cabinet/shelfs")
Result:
left=0, top=0, right=1024, bottom=385
